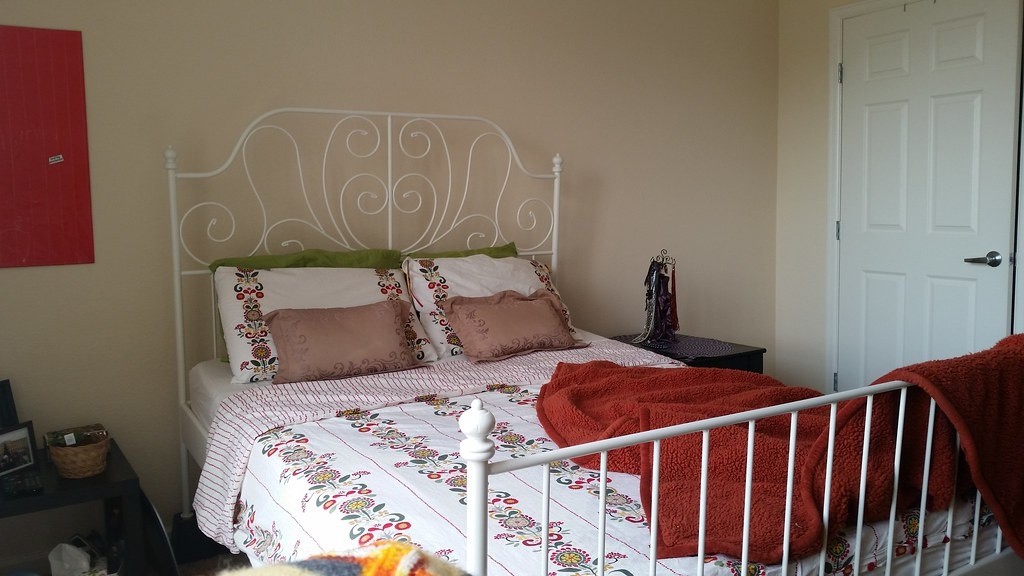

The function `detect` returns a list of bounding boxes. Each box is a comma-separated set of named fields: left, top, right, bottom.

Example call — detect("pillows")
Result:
left=440, top=287, right=591, bottom=363
left=213, top=265, right=440, bottom=383
left=211, top=249, right=401, bottom=365
left=404, top=242, right=521, bottom=261
left=401, top=253, right=585, bottom=361
left=262, top=300, right=433, bottom=383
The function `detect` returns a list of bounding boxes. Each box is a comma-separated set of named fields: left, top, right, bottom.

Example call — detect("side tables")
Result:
left=609, top=332, right=769, bottom=379
left=0, top=436, right=142, bottom=575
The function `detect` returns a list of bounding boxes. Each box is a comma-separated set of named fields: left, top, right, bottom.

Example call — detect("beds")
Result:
left=177, top=323, right=1023, bottom=575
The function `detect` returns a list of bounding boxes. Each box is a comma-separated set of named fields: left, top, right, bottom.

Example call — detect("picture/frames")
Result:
left=1, top=420, right=40, bottom=484
